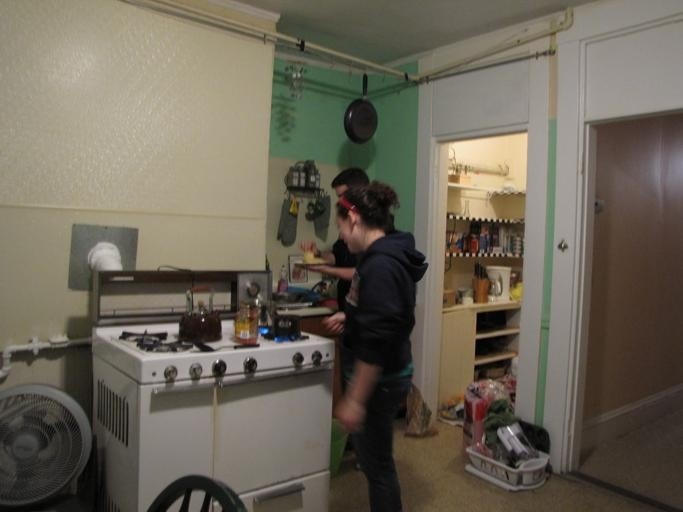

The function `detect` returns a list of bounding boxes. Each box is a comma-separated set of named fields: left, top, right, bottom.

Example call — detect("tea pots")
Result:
left=179, top=301, right=222, bottom=343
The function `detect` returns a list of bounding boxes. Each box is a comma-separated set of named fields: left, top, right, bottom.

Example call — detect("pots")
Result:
left=343, top=73, right=378, bottom=145
left=272, top=314, right=303, bottom=336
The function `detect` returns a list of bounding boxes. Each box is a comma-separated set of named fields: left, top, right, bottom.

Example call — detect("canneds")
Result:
left=235, top=303, right=258, bottom=344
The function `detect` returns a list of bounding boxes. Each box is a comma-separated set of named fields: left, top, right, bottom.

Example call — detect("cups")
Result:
left=472, top=279, right=489, bottom=303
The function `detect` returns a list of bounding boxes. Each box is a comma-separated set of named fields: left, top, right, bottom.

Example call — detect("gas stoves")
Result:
left=92, top=315, right=336, bottom=384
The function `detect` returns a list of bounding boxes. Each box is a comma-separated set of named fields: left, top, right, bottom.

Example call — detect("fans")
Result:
left=0, top=381, right=94, bottom=508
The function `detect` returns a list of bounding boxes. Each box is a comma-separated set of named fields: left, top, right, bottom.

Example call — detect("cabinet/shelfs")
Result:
left=444, top=212, right=522, bottom=258
left=437, top=304, right=519, bottom=412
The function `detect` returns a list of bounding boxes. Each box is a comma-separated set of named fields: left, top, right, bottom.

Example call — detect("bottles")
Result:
left=289, top=160, right=320, bottom=188
left=471, top=233, right=486, bottom=253
left=278, top=265, right=288, bottom=292
left=236, top=300, right=259, bottom=345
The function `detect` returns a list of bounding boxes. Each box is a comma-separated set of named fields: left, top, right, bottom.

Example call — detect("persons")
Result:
left=320, top=181, right=430, bottom=511
left=295, top=166, right=396, bottom=281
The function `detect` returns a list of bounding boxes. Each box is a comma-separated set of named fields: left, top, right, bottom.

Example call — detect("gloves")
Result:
left=277, top=198, right=299, bottom=246
